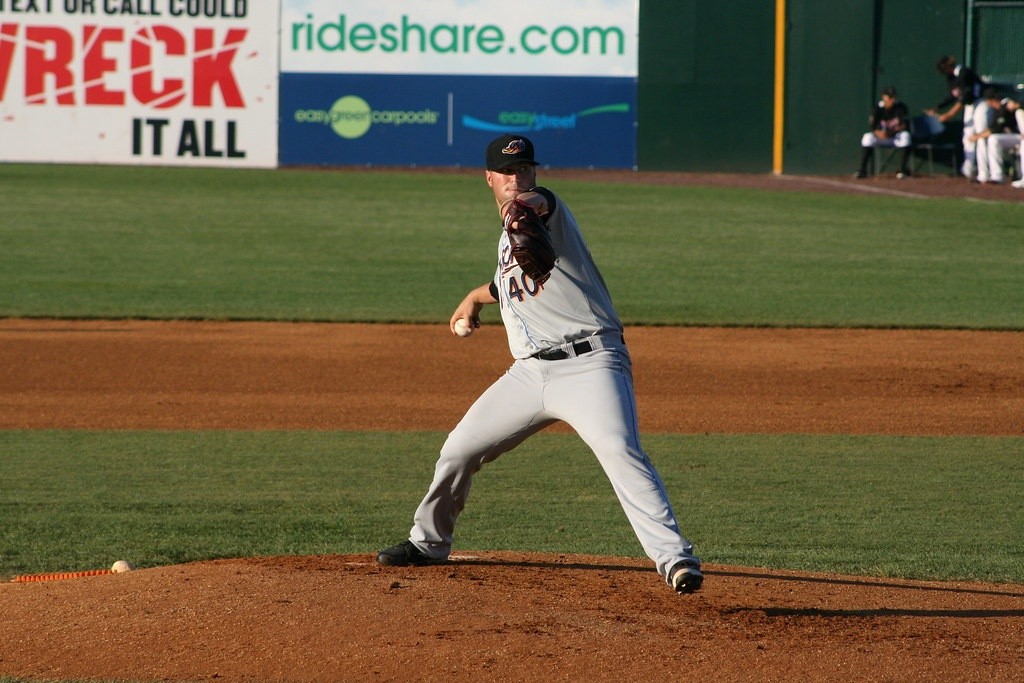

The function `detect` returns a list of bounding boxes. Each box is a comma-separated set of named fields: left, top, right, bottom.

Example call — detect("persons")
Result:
left=376, top=132, right=703, bottom=591
left=924, top=55, right=1024, bottom=188
left=850, top=86, right=912, bottom=181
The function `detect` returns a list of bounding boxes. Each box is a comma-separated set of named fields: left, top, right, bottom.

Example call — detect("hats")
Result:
left=486, top=133, right=540, bottom=171
left=936, top=54, right=951, bottom=74
left=881, top=84, right=897, bottom=98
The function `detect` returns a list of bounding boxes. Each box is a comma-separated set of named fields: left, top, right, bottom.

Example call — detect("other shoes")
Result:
left=895, top=168, right=906, bottom=179
left=854, top=169, right=866, bottom=178
left=1011, top=179, right=1024, bottom=189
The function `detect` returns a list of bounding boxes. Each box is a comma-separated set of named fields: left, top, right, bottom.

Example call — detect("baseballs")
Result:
left=454, top=318, right=475, bottom=338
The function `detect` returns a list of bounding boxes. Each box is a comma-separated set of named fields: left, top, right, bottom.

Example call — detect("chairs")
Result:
left=868, top=114, right=960, bottom=179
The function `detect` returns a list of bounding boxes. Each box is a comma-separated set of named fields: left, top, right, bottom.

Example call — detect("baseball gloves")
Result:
left=501, top=198, right=557, bottom=286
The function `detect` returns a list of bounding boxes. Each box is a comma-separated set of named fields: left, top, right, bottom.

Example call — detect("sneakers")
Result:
left=377, top=541, right=447, bottom=564
left=669, top=561, right=703, bottom=595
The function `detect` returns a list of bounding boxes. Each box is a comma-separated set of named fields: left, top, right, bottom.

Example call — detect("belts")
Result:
left=532, top=335, right=626, bottom=360
